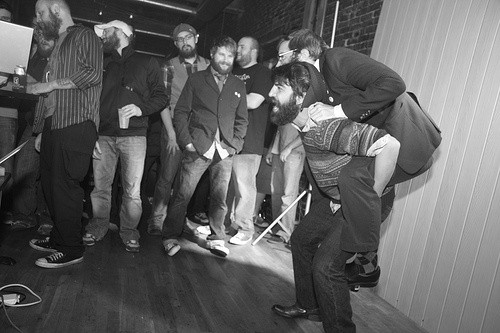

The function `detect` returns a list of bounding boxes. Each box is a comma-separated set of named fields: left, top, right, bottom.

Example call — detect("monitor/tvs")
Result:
left=0, top=20, right=34, bottom=78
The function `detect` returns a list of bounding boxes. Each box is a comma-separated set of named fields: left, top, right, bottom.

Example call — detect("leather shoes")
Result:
left=346, top=262, right=380, bottom=291
left=271, top=302, right=322, bottom=322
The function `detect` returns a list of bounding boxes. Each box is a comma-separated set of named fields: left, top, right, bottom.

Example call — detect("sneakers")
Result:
left=30, top=236, right=58, bottom=252
left=34, top=250, right=84, bottom=268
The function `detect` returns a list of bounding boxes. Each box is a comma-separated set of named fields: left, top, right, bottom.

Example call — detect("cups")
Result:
left=118, top=108, right=129, bottom=129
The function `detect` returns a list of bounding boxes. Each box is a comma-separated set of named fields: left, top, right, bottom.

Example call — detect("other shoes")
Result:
left=254, top=215, right=268, bottom=227
left=268, top=235, right=285, bottom=244
left=125, top=239, right=140, bottom=252
left=83, top=232, right=95, bottom=246
left=148, top=224, right=162, bottom=235
left=163, top=242, right=181, bottom=256
left=37, top=223, right=53, bottom=235
left=191, top=212, right=209, bottom=225
left=205, top=240, right=228, bottom=257
left=229, top=232, right=252, bottom=244
left=5, top=217, right=36, bottom=230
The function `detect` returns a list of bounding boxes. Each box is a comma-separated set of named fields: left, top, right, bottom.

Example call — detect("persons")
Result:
left=81, top=19, right=168, bottom=254
left=0, top=1, right=106, bottom=268
left=267, top=62, right=396, bottom=333
left=259, top=121, right=308, bottom=243
left=147, top=22, right=273, bottom=257
left=275, top=27, right=442, bottom=287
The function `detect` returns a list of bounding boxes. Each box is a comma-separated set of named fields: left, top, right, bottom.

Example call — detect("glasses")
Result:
left=173, top=33, right=195, bottom=42
left=275, top=48, right=296, bottom=59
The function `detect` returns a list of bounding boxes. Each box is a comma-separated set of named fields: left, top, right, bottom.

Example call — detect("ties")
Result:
left=215, top=74, right=226, bottom=91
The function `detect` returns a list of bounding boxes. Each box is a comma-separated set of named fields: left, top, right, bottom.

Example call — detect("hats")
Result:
left=172, top=22, right=199, bottom=41
left=94, top=20, right=134, bottom=38
